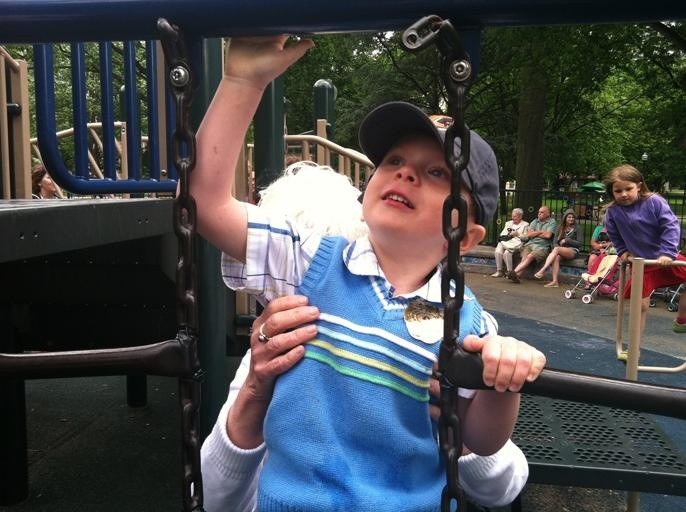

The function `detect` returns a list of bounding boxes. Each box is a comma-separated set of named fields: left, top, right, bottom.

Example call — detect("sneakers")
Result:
left=491, top=271, right=502, bottom=278
left=512, top=271, right=521, bottom=284
left=505, top=272, right=511, bottom=278
left=619, top=350, right=642, bottom=362
left=672, top=318, right=686, bottom=333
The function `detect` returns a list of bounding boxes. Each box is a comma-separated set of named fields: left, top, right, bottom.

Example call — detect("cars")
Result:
left=501, top=175, right=610, bottom=221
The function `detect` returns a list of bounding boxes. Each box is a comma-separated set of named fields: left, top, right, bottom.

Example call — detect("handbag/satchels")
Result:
left=498, top=228, right=515, bottom=241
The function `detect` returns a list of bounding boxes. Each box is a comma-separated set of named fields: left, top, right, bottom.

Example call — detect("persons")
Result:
left=601, top=164, right=685, bottom=361
left=28, top=166, right=58, bottom=199
left=491, top=205, right=611, bottom=288
left=200, top=162, right=531, bottom=511
left=183, top=34, right=547, bottom=510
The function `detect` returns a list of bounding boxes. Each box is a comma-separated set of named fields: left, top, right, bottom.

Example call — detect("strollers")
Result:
left=647, top=282, right=686, bottom=313
left=560, top=239, right=620, bottom=304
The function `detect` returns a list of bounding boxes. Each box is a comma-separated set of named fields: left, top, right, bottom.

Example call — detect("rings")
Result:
left=257, top=325, right=270, bottom=345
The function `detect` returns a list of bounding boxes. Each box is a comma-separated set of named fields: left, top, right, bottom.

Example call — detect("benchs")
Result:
left=460, top=244, right=589, bottom=285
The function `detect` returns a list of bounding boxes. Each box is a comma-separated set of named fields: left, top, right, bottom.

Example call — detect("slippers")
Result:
left=535, top=274, right=545, bottom=279
left=544, top=282, right=559, bottom=288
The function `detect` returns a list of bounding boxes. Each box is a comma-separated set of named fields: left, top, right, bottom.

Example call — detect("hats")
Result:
left=357, top=101, right=502, bottom=228
